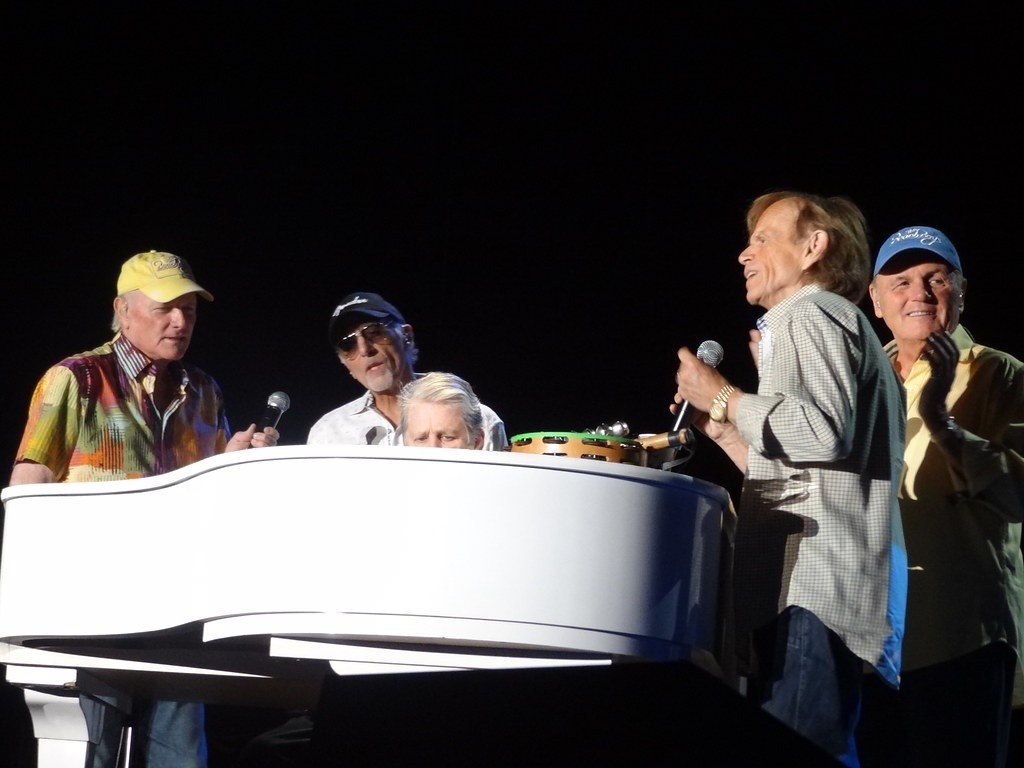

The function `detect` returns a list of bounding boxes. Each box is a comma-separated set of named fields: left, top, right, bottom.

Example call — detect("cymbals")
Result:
left=515, top=435, right=640, bottom=461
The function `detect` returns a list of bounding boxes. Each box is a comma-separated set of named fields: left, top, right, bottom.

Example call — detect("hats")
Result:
left=873, top=226, right=963, bottom=276
left=331, top=293, right=404, bottom=345
left=116, top=250, right=214, bottom=304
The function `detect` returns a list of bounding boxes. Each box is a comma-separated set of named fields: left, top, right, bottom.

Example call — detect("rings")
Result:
left=677, top=372, right=680, bottom=376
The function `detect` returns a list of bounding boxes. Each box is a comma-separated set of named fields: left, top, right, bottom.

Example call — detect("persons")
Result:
left=671, top=189, right=910, bottom=759
left=8, top=248, right=278, bottom=768
left=303, top=292, right=508, bottom=451
left=232, top=372, right=485, bottom=768
left=866, top=225, right=1024, bottom=768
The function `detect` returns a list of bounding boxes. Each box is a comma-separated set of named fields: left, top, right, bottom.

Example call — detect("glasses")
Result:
left=334, top=319, right=395, bottom=359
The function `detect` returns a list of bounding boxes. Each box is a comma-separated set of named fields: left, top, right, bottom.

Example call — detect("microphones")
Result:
left=673, top=340, right=724, bottom=432
left=248, top=391, right=290, bottom=449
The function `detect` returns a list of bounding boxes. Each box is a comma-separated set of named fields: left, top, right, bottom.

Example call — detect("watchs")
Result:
left=927, top=414, right=956, bottom=448
left=707, top=384, right=735, bottom=421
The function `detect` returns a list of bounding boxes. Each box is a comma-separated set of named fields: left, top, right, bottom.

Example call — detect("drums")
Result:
left=509, top=430, right=649, bottom=468
